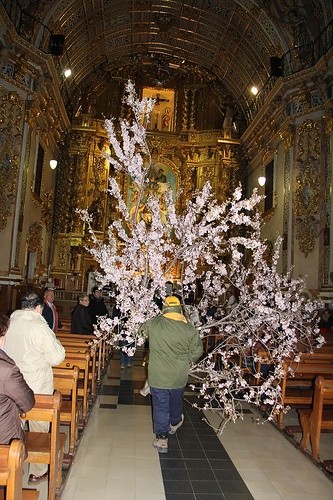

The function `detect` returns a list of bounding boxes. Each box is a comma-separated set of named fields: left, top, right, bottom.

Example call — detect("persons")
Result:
left=113, top=292, right=139, bottom=368
left=138, top=297, right=203, bottom=453
left=88, top=287, right=108, bottom=353
left=70, top=294, right=94, bottom=336
left=85, top=262, right=203, bottom=308
left=204, top=277, right=332, bottom=402
left=41, top=290, right=65, bottom=333
left=0, top=313, right=35, bottom=488
left=3, top=291, right=65, bottom=482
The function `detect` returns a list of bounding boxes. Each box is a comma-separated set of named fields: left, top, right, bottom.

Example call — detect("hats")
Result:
left=164, top=295, right=180, bottom=307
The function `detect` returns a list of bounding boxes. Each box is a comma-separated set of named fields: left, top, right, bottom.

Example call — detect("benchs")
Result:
left=204, top=321, right=333, bottom=461
left=0, top=319, right=113, bottom=500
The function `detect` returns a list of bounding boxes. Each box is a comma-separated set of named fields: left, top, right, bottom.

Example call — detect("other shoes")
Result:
left=120, top=363, right=126, bottom=368
left=127, top=362, right=133, bottom=367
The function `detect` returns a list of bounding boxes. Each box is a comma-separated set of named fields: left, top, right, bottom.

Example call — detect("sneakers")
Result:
left=153, top=438, right=168, bottom=453
left=168, top=414, right=184, bottom=435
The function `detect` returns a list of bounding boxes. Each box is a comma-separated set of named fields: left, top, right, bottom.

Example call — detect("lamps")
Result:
left=16, top=0, right=67, bottom=56
left=268, top=22, right=333, bottom=78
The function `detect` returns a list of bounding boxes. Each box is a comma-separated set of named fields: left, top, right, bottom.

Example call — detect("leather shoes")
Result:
left=27, top=471, right=48, bottom=486
